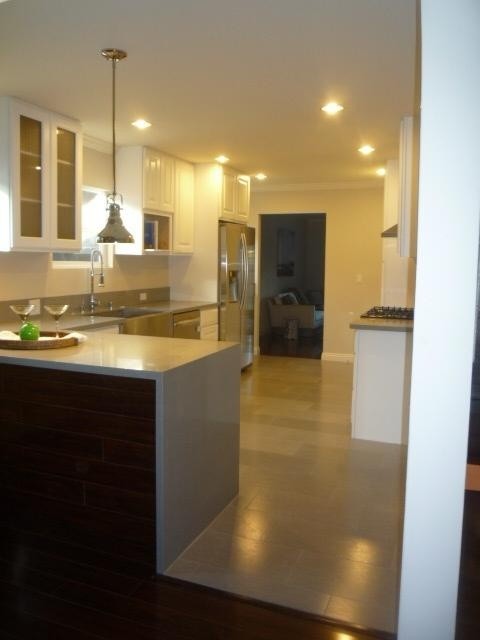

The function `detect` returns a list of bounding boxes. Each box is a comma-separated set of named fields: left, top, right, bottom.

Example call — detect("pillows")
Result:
left=273, top=288, right=309, bottom=305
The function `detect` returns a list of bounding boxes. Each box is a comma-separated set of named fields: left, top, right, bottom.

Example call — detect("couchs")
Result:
left=265, top=290, right=323, bottom=337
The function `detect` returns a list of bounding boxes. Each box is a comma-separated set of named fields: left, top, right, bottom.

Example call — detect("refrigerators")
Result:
left=219, top=222, right=255, bottom=371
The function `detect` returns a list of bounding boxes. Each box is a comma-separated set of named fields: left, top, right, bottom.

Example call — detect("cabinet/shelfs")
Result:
left=196, top=162, right=251, bottom=224
left=0, top=95, right=83, bottom=252
left=115, top=145, right=195, bottom=255
left=200, top=307, right=218, bottom=342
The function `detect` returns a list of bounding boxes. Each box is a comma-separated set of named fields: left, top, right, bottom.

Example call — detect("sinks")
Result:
left=79, top=306, right=165, bottom=319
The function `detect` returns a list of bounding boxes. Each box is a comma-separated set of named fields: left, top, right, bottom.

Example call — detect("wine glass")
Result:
left=44, top=302, right=71, bottom=338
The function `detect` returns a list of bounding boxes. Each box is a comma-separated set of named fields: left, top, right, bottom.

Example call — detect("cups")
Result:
left=9, top=305, right=35, bottom=323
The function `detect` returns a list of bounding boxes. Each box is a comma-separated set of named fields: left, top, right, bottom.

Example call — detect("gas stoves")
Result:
left=360, top=305, right=414, bottom=320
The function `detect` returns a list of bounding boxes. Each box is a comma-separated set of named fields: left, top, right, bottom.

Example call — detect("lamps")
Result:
left=97, top=46, right=135, bottom=239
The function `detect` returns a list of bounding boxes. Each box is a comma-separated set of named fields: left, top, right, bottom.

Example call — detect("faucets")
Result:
left=89, top=249, right=105, bottom=307
